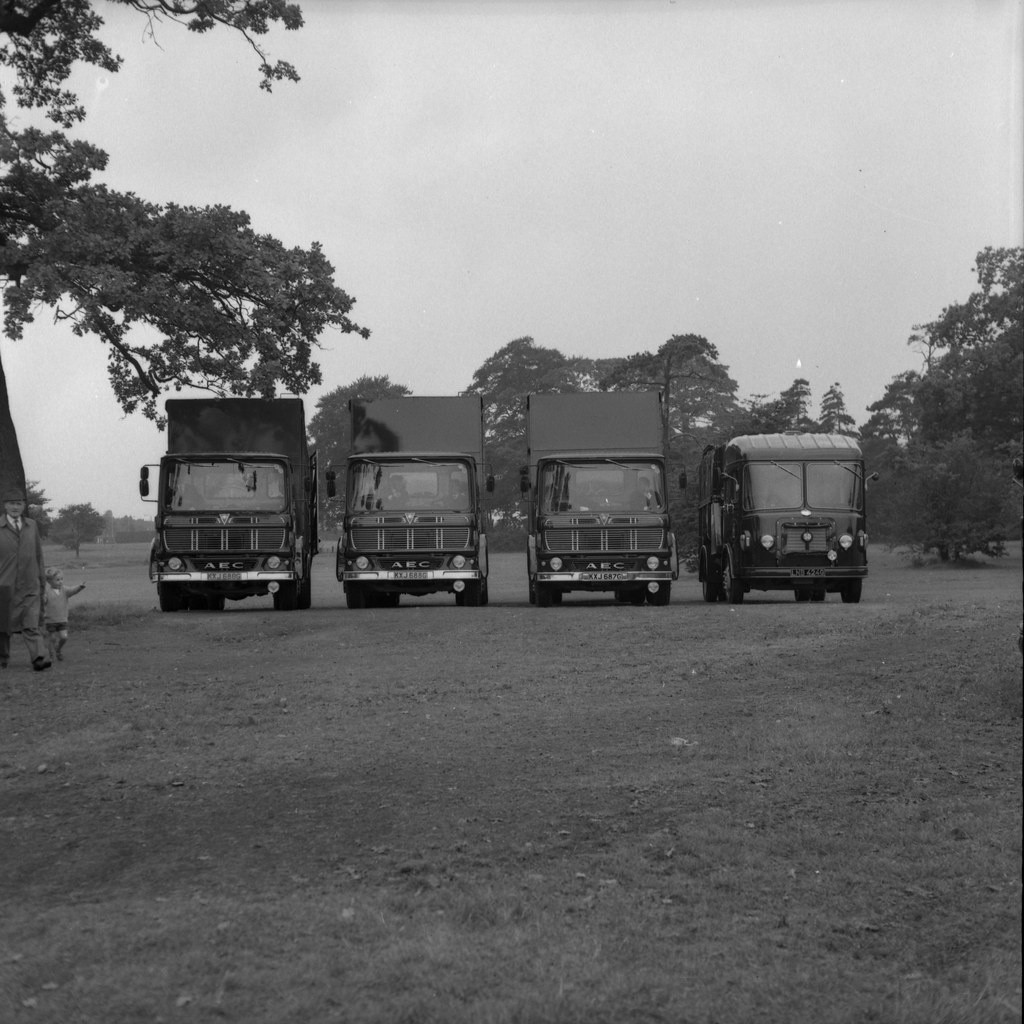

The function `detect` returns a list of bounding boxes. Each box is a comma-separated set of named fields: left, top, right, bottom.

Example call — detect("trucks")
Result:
left=327, top=394, right=495, bottom=606
left=697, top=433, right=879, bottom=602
left=517, top=392, right=685, bottom=605
left=141, top=397, right=320, bottom=610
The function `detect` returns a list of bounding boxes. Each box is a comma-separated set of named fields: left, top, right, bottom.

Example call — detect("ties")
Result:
left=13, top=519, right=20, bottom=530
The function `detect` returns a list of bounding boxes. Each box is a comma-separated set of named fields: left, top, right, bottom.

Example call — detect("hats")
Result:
left=3, top=489, right=28, bottom=502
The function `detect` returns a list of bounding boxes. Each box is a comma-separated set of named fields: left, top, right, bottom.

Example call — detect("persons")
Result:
left=40, top=566, right=88, bottom=660
left=626, top=477, right=663, bottom=512
left=441, top=477, right=466, bottom=508
left=378, top=473, right=410, bottom=508
left=0, top=484, right=52, bottom=671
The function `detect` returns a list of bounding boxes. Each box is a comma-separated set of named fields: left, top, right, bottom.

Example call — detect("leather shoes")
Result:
left=33, top=660, right=52, bottom=672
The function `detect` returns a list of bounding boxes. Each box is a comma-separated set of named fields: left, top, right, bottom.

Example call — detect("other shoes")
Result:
left=49, top=652, right=55, bottom=661
left=55, top=649, right=64, bottom=660
